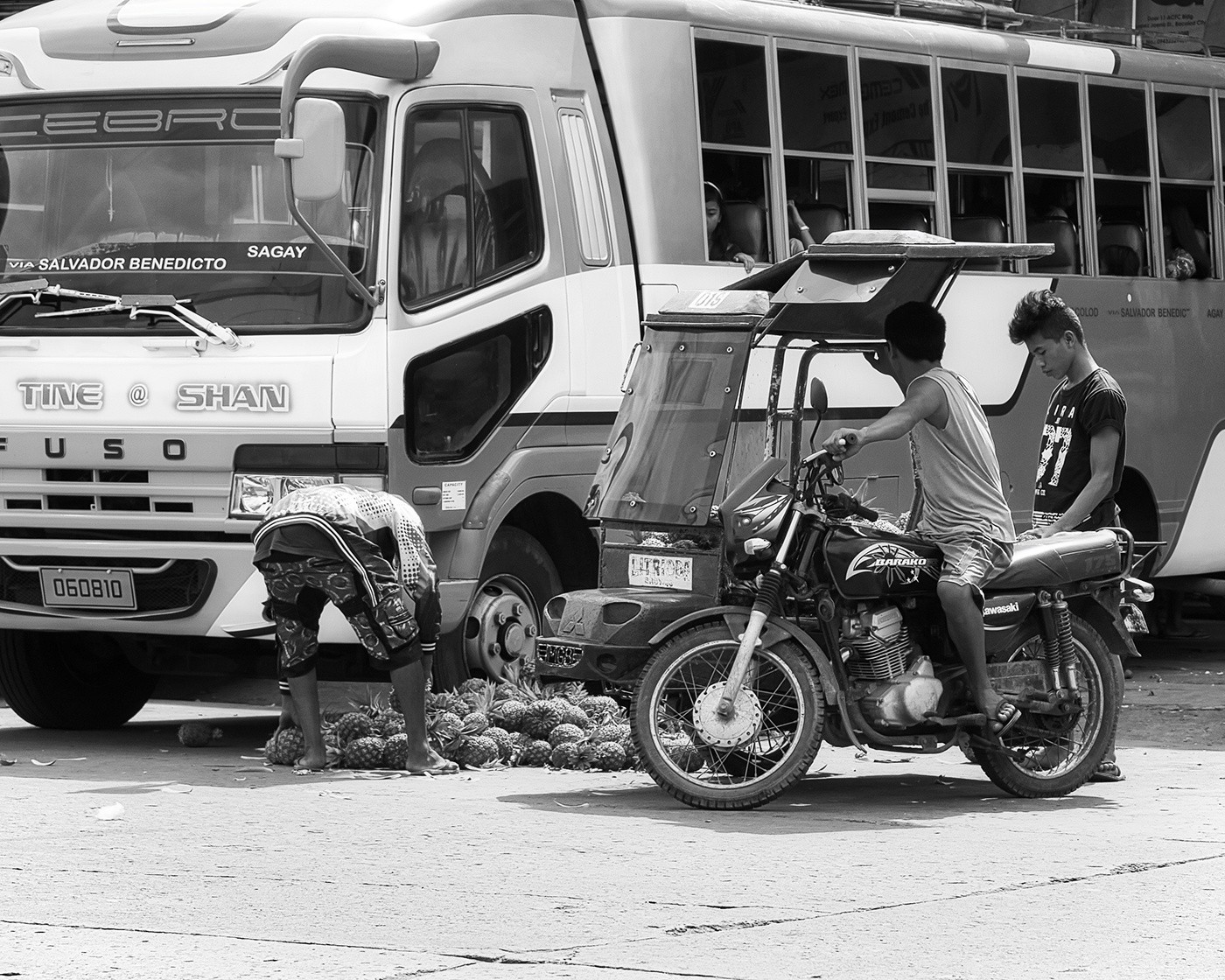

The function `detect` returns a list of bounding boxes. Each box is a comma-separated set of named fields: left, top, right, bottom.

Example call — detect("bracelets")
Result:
left=799, top=225, right=810, bottom=231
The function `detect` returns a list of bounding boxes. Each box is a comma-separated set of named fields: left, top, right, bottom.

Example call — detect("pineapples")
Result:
left=859, top=510, right=913, bottom=535
left=178, top=722, right=223, bottom=748
left=266, top=665, right=704, bottom=772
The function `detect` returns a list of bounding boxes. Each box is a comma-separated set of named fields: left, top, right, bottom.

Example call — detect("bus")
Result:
left=2, top=0, right=1225, bottom=730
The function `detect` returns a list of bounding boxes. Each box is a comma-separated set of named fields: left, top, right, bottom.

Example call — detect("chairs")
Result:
left=951, top=216, right=1009, bottom=272
left=1096, top=221, right=1149, bottom=276
left=870, top=209, right=930, bottom=236
left=789, top=205, right=847, bottom=253
left=399, top=136, right=505, bottom=305
left=1171, top=226, right=1213, bottom=279
left=1026, top=214, right=1080, bottom=274
left=717, top=200, right=767, bottom=263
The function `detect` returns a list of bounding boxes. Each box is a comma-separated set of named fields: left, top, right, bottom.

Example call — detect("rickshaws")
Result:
left=530, top=231, right=1125, bottom=798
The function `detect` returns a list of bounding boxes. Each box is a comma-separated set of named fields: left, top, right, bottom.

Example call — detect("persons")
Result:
left=1008, top=288, right=1126, bottom=783
left=1046, top=183, right=1199, bottom=280
left=758, top=194, right=815, bottom=256
left=821, top=300, right=1024, bottom=738
left=253, top=483, right=460, bottom=775
left=703, top=181, right=754, bottom=274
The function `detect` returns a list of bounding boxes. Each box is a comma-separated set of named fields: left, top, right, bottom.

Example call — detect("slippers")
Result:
left=293, top=757, right=323, bottom=772
left=412, top=759, right=459, bottom=775
left=987, top=699, right=1022, bottom=736
left=1087, top=760, right=1122, bottom=782
